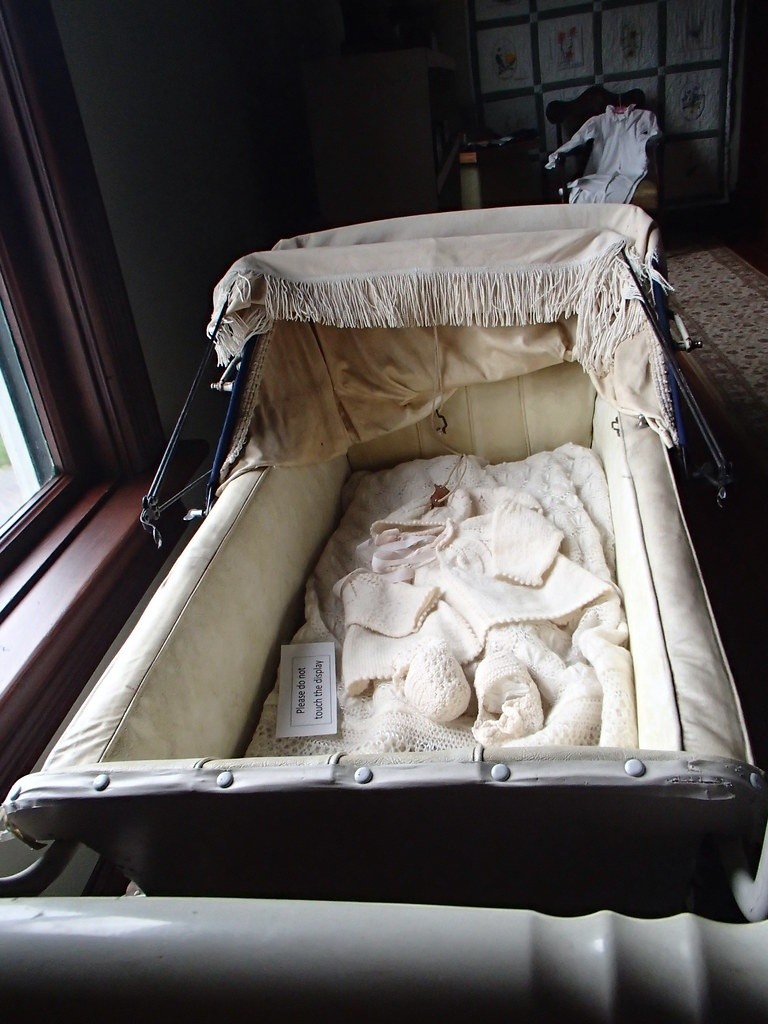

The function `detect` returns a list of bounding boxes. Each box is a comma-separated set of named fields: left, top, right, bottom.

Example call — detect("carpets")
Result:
left=664, top=243, right=768, bottom=464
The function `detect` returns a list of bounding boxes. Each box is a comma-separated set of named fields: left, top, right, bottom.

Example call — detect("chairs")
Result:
left=546, top=86, right=658, bottom=222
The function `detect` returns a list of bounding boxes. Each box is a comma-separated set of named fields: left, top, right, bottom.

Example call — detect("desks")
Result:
left=440, top=132, right=500, bottom=211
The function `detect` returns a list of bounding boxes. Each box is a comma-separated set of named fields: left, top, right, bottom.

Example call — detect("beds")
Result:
left=0, top=202, right=768, bottom=925
